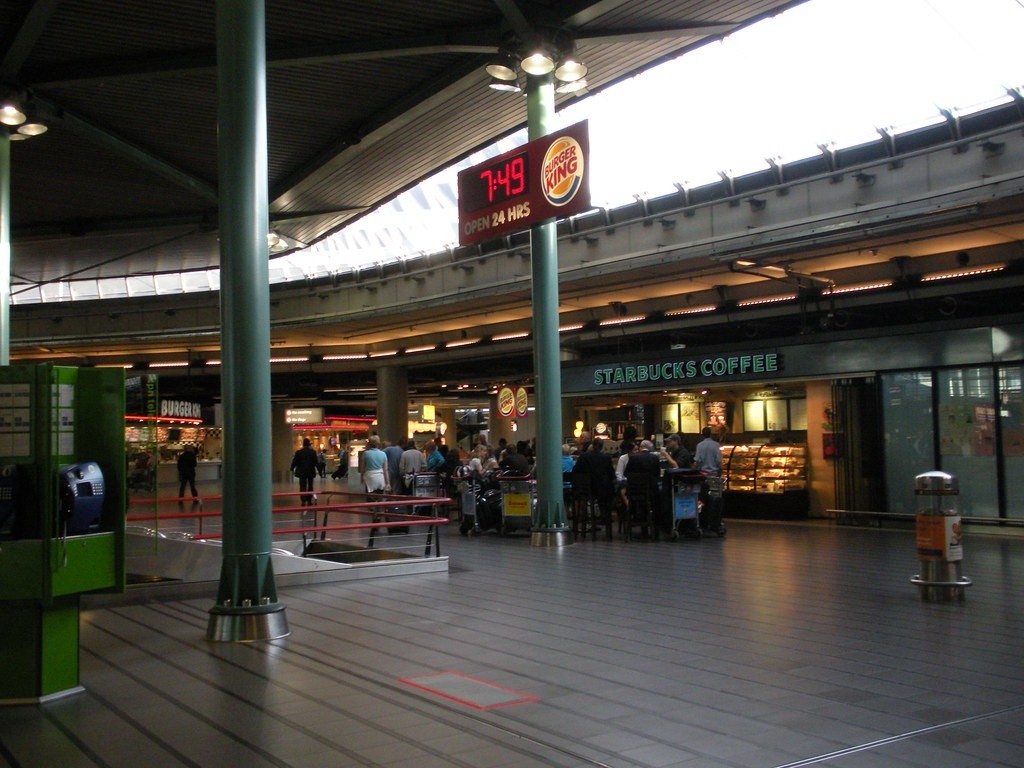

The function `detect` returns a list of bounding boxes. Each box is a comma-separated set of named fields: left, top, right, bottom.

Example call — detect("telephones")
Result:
left=52, top=461, right=105, bottom=532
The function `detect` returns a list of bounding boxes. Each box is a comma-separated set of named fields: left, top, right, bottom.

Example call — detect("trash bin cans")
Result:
left=908, top=470, right=974, bottom=602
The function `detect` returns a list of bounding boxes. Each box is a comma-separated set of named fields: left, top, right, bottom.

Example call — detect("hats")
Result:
left=663, top=433, right=681, bottom=441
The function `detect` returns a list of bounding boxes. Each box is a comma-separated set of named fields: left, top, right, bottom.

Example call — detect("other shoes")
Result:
left=592, top=525, right=602, bottom=531
left=459, top=527, right=469, bottom=536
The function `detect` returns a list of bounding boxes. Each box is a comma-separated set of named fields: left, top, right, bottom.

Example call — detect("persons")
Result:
left=320, top=449, right=327, bottom=478
left=360, top=426, right=726, bottom=543
left=177, top=444, right=199, bottom=504
left=290, top=438, right=322, bottom=507
